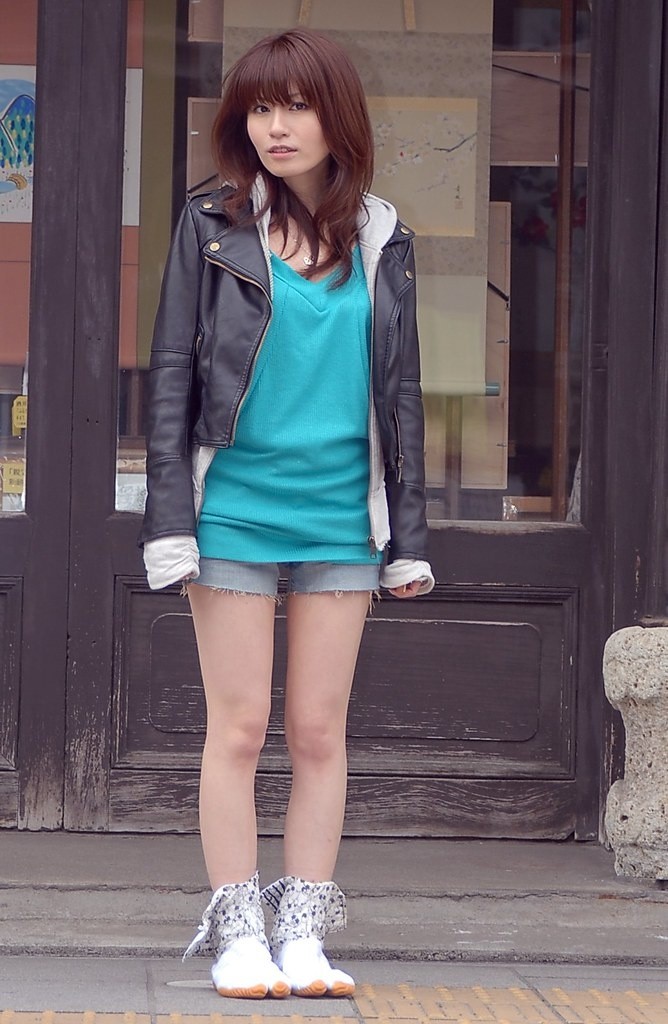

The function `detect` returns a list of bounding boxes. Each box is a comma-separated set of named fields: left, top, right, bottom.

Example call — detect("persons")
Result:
left=138, top=28, right=437, bottom=997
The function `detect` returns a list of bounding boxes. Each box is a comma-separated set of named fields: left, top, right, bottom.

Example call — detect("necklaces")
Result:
left=288, top=225, right=312, bottom=265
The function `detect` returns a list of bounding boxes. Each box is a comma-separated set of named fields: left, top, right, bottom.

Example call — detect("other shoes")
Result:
left=260, top=876, right=356, bottom=999
left=182, top=871, right=293, bottom=999
